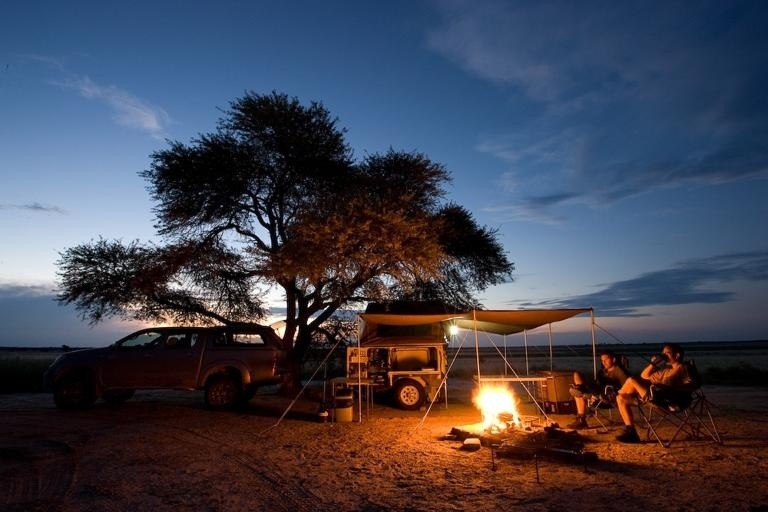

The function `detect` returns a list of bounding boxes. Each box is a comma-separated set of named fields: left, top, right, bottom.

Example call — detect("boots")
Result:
left=615, top=427, right=640, bottom=443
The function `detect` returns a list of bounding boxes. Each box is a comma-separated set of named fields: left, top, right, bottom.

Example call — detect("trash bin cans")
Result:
left=335, top=388, right=354, bottom=421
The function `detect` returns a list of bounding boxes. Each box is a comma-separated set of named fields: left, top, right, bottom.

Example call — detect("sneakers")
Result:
left=567, top=415, right=588, bottom=429
left=569, top=384, right=593, bottom=399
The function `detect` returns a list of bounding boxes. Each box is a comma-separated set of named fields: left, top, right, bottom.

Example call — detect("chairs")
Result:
left=165, top=338, right=191, bottom=350
left=583, top=354, right=629, bottom=433
left=638, top=359, right=724, bottom=448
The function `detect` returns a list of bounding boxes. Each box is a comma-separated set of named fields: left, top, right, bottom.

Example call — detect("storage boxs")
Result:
left=335, top=389, right=353, bottom=422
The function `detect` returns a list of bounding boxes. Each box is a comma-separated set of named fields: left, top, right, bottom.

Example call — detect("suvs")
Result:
left=48, top=322, right=290, bottom=411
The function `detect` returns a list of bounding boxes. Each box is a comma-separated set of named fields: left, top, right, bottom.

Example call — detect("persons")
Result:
left=615, top=343, right=690, bottom=443
left=566, top=348, right=628, bottom=430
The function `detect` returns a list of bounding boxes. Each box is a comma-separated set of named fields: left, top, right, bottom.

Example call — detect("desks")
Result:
left=473, top=374, right=547, bottom=416
